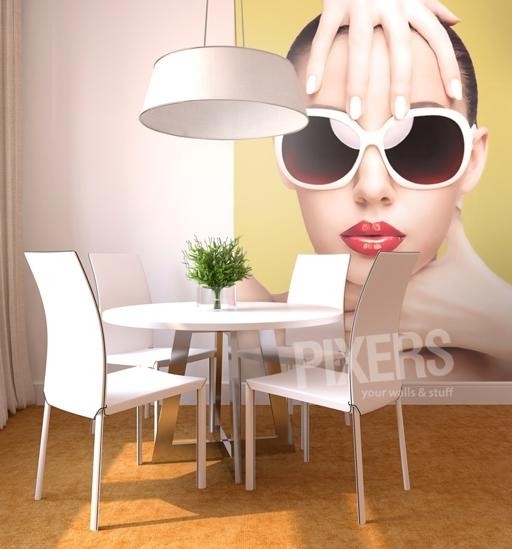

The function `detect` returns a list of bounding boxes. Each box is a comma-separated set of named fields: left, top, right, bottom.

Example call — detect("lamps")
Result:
left=138, top=0, right=309, bottom=141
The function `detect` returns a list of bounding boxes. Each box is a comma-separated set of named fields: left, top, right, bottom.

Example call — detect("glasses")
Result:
left=269, top=101, right=484, bottom=193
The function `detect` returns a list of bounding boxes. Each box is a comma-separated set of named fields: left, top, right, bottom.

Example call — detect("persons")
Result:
left=236, top=2, right=512, bottom=382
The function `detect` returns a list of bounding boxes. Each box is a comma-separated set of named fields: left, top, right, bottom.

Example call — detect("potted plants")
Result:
left=181, top=234, right=257, bottom=310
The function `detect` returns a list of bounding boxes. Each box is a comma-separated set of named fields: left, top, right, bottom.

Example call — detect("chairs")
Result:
left=231, top=252, right=352, bottom=450
left=22, top=249, right=209, bottom=531
left=86, top=247, right=220, bottom=442
left=245, top=250, right=421, bottom=527
